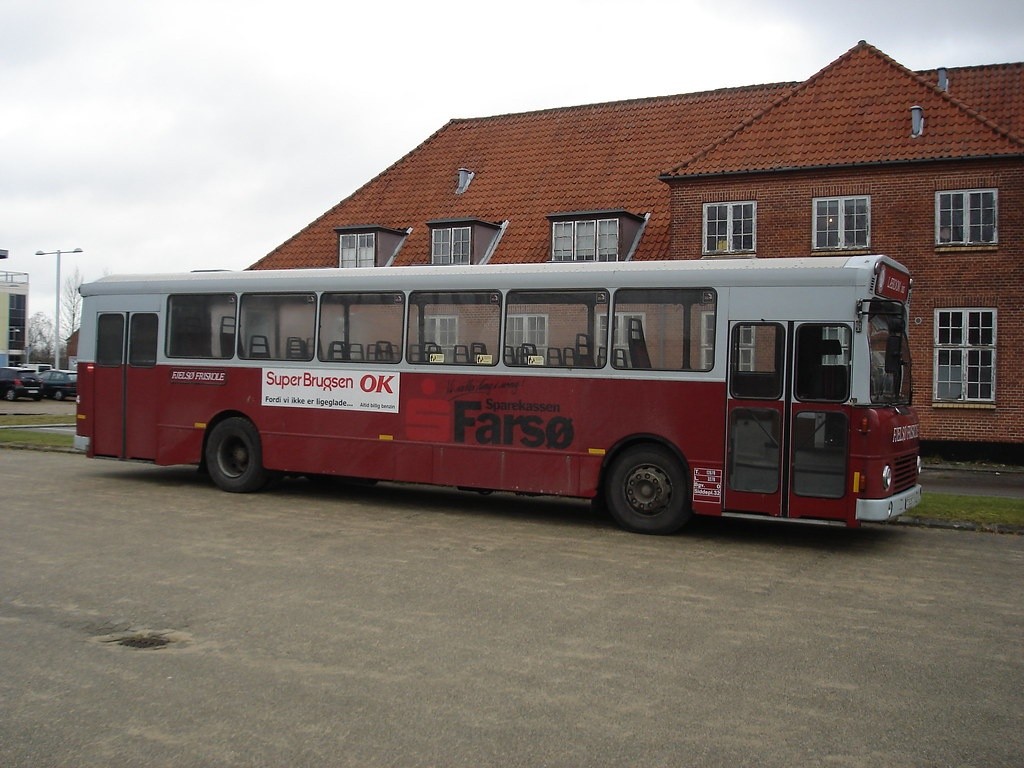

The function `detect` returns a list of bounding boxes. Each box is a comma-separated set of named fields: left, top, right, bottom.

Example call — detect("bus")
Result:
left=72, top=253, right=926, bottom=534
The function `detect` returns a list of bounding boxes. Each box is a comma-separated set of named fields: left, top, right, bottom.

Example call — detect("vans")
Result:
left=20, top=363, right=53, bottom=377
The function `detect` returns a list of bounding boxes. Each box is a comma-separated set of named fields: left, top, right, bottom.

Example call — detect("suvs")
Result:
left=0, top=367, right=45, bottom=401
left=38, top=368, right=79, bottom=401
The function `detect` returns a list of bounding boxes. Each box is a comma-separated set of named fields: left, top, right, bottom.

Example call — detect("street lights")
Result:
left=35, top=247, right=83, bottom=369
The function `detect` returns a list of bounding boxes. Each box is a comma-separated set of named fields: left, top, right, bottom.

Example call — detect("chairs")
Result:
left=249, top=333, right=627, bottom=370
left=628, top=317, right=652, bottom=369
left=219, top=316, right=245, bottom=359
left=185, top=317, right=203, bottom=356
left=733, top=371, right=781, bottom=398
left=809, top=340, right=846, bottom=399
